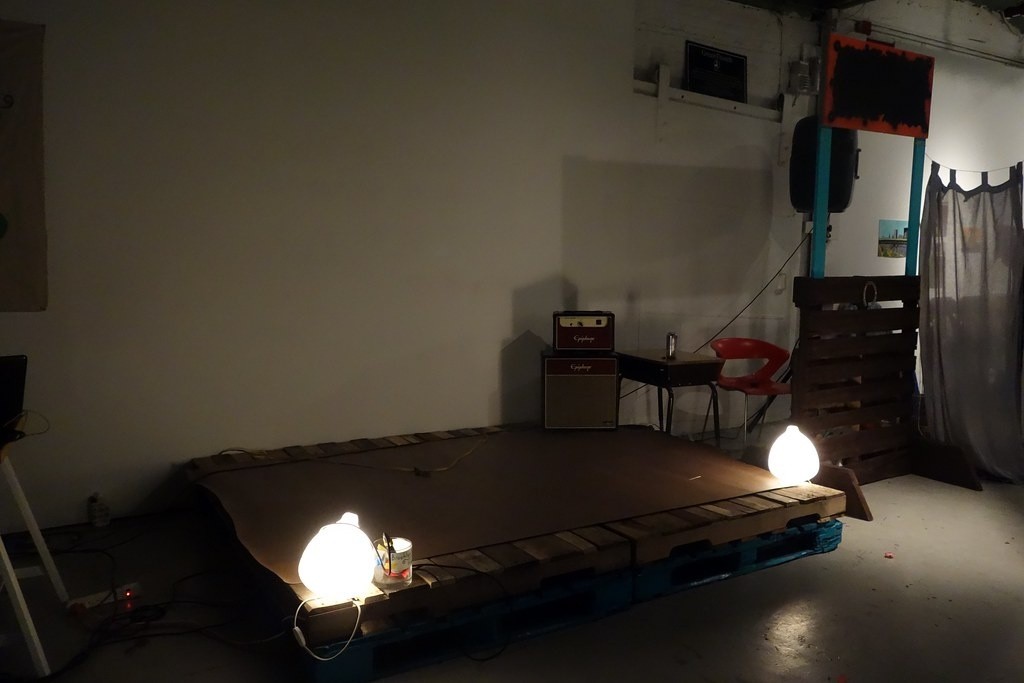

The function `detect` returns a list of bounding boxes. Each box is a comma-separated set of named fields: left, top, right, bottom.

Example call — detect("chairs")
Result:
left=702, top=338, right=791, bottom=448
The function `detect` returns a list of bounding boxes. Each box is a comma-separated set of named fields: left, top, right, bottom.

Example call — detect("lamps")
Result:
left=768, top=423, right=820, bottom=484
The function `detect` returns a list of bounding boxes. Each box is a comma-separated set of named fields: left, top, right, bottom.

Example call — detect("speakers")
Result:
left=541, top=350, right=620, bottom=430
left=552, top=311, right=614, bottom=352
left=683, top=40, right=747, bottom=104
left=789, top=116, right=858, bottom=214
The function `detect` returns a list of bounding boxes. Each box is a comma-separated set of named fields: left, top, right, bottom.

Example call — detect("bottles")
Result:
left=666, top=331, right=677, bottom=360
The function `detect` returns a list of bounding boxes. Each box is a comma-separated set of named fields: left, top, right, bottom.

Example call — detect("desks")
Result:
left=614, top=349, right=726, bottom=448
left=0, top=407, right=69, bottom=678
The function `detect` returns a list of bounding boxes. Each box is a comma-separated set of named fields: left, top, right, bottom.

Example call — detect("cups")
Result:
left=373, top=537, right=413, bottom=589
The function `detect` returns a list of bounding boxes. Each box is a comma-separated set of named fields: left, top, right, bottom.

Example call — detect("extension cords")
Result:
left=66, top=582, right=143, bottom=612
